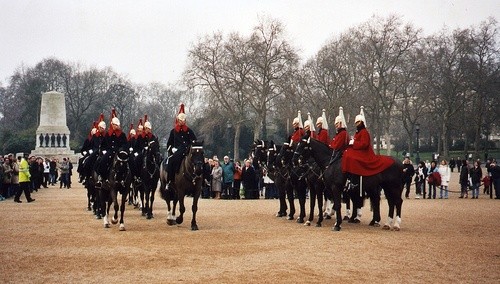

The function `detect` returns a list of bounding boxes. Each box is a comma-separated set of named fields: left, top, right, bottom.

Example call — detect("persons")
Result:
left=57, top=133, right=61, bottom=147
left=165, top=104, right=202, bottom=190
left=45, top=133, right=49, bottom=147
left=304, top=111, right=316, bottom=139
left=329, top=106, right=350, bottom=153
left=414, top=158, right=456, bottom=199
left=77, top=107, right=160, bottom=189
left=431, top=152, right=440, bottom=163
left=312, top=108, right=330, bottom=146
left=203, top=155, right=274, bottom=200
left=401, top=157, right=413, bottom=199
left=287, top=110, right=304, bottom=145
left=341, top=106, right=396, bottom=194
left=59, top=157, right=73, bottom=189
left=51, top=132, right=55, bottom=147
left=480, top=150, right=500, bottom=199
left=62, top=133, right=67, bottom=147
left=39, top=133, right=43, bottom=147
left=457, top=153, right=481, bottom=199
left=0, top=153, right=59, bottom=203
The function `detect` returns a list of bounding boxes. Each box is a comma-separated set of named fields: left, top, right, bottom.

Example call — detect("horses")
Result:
left=293, top=137, right=404, bottom=231
left=159, top=138, right=204, bottom=231
left=129, top=137, right=159, bottom=219
left=253, top=139, right=358, bottom=227
left=86, top=150, right=129, bottom=231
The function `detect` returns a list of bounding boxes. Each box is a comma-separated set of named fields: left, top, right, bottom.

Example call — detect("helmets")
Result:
left=88, top=108, right=121, bottom=140
left=355, top=105, right=367, bottom=128
left=127, top=115, right=152, bottom=141
left=335, top=106, right=347, bottom=128
left=316, top=109, right=329, bottom=129
left=304, top=112, right=316, bottom=131
left=293, top=111, right=303, bottom=128
left=176, top=104, right=186, bottom=126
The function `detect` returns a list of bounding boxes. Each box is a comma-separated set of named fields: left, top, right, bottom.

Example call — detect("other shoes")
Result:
left=27, top=198, right=35, bottom=202
left=14, top=200, right=22, bottom=203
